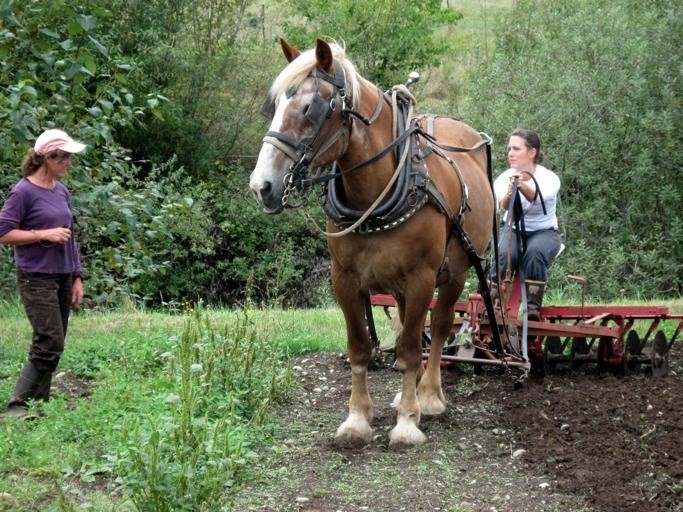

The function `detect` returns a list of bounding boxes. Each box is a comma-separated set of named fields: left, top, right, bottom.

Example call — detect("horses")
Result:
left=248, top=34, right=496, bottom=451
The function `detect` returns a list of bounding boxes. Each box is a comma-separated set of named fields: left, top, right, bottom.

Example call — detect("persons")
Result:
left=0, top=128, right=86, bottom=427
left=478, top=127, right=561, bottom=320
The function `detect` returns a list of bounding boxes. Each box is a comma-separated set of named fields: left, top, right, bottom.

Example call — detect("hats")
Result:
left=31, top=127, right=88, bottom=157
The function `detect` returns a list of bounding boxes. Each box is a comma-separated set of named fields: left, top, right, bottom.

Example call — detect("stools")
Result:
left=548, top=242, right=565, bottom=273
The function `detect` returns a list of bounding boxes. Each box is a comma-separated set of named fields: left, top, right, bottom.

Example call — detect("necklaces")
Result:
left=35, top=175, right=55, bottom=193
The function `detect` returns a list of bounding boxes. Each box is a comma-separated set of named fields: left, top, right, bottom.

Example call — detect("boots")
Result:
left=521, top=278, right=547, bottom=321
left=481, top=278, right=499, bottom=317
